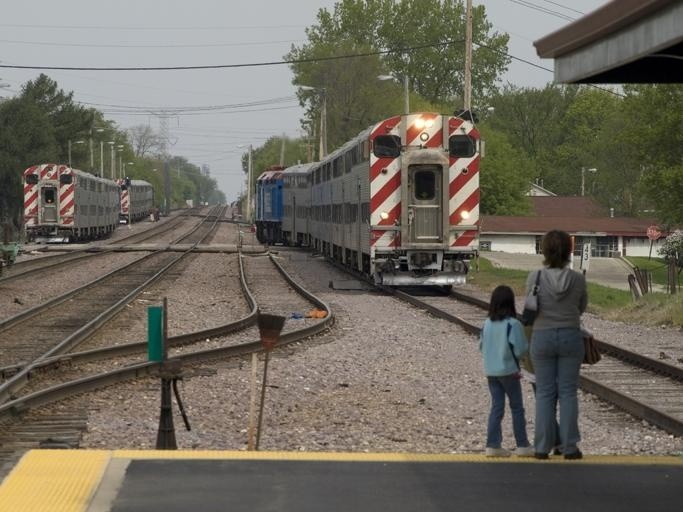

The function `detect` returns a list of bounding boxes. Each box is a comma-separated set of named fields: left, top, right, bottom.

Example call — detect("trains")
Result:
left=21, top=162, right=122, bottom=246
left=110, top=179, right=154, bottom=224
left=253, top=111, right=483, bottom=295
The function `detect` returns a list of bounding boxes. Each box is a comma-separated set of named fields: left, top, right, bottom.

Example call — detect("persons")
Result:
left=521, top=229, right=588, bottom=459
left=478, top=285, right=534, bottom=457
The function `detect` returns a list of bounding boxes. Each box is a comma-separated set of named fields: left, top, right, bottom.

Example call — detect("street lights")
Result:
left=67, top=140, right=84, bottom=168
left=89, top=128, right=103, bottom=168
left=110, top=144, right=135, bottom=179
left=300, top=84, right=327, bottom=157
left=100, top=141, right=114, bottom=179
left=462, top=106, right=494, bottom=122
left=377, top=72, right=409, bottom=114
left=236, top=144, right=252, bottom=224
left=580, top=166, right=597, bottom=197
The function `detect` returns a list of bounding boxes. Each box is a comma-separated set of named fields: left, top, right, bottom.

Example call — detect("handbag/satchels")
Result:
left=583, top=335, right=602, bottom=365
left=521, top=291, right=539, bottom=327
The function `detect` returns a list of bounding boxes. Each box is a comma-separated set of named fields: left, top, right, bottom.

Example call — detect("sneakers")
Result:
left=484, top=444, right=582, bottom=459
